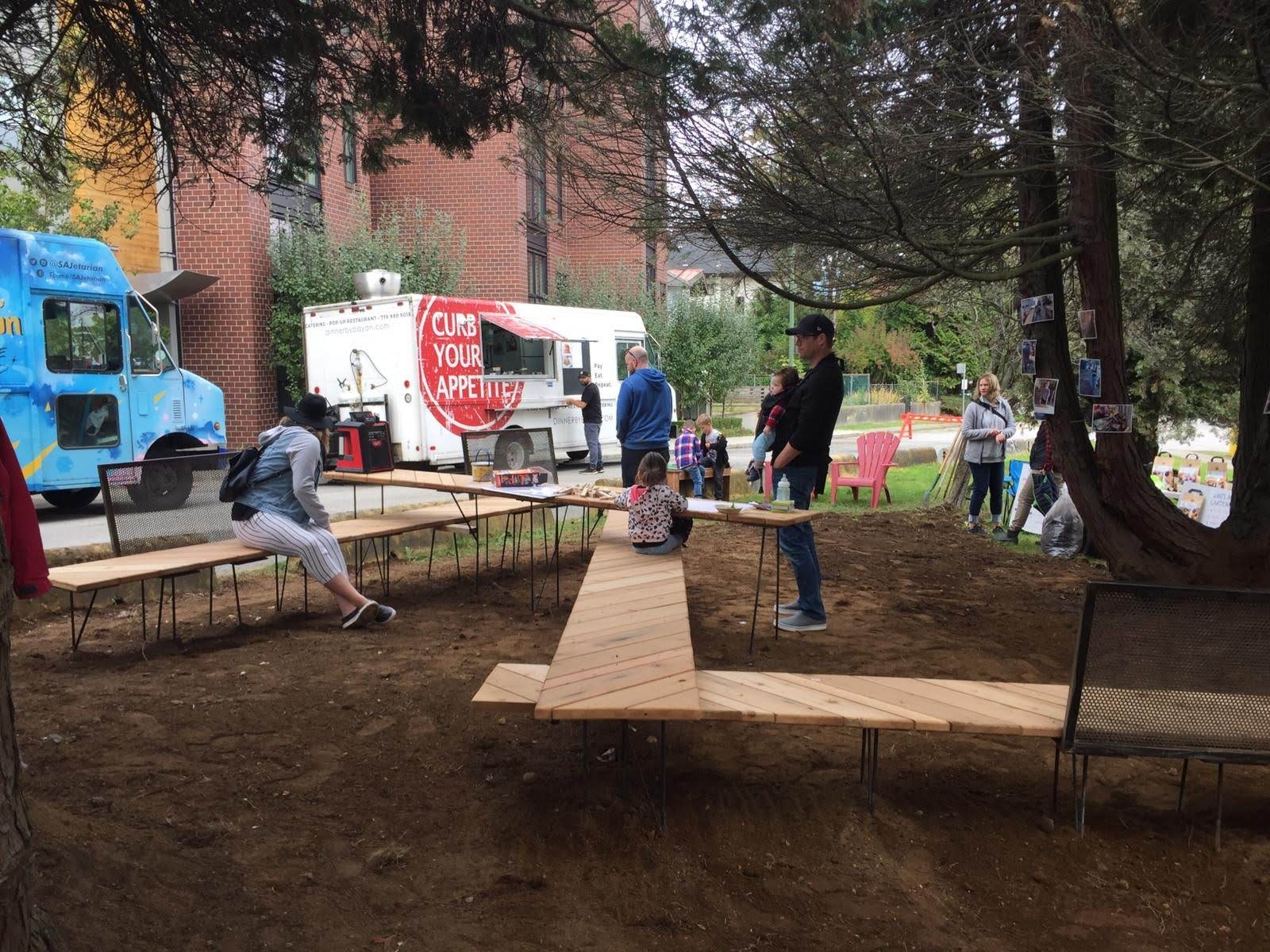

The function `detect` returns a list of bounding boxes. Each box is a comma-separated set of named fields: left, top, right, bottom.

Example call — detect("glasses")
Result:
left=793, top=333, right=820, bottom=343
left=578, top=376, right=586, bottom=378
left=624, top=349, right=638, bottom=367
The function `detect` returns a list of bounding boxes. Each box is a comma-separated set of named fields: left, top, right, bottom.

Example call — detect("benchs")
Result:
left=48, top=496, right=556, bottom=650
left=470, top=665, right=1270, bottom=850
left=667, top=468, right=731, bottom=503
left=533, top=509, right=701, bottom=839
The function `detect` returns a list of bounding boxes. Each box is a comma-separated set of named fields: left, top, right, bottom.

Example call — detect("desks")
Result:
left=324, top=468, right=823, bottom=657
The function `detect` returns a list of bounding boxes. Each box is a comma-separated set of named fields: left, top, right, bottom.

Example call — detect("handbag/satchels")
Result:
left=1205, top=456, right=1227, bottom=488
left=1177, top=453, right=1201, bottom=485
left=1151, top=451, right=1174, bottom=490
left=1179, top=488, right=1205, bottom=520
left=1041, top=484, right=1084, bottom=562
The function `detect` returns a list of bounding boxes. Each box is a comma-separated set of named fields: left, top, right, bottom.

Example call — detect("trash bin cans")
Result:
left=844, top=374, right=869, bottom=396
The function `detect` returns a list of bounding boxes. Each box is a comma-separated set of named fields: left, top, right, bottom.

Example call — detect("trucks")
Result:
left=2, top=228, right=229, bottom=512
left=303, top=270, right=684, bottom=477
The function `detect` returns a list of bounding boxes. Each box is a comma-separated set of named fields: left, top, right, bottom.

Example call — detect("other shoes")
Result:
left=578, top=466, right=605, bottom=474
left=340, top=598, right=397, bottom=630
left=745, top=460, right=762, bottom=482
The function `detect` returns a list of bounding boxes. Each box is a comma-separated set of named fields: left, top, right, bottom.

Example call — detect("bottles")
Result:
left=777, top=473, right=790, bottom=500
left=531, top=368, right=544, bottom=374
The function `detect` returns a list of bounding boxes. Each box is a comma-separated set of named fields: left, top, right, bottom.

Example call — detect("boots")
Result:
left=994, top=528, right=1021, bottom=544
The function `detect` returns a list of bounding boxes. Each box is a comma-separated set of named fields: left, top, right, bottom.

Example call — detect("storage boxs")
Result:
left=491, top=466, right=551, bottom=488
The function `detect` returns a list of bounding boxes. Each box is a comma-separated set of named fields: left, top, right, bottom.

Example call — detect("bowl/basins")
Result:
left=715, top=505, right=743, bottom=514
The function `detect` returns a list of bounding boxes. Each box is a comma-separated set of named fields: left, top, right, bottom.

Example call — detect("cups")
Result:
left=520, top=368, right=527, bottom=375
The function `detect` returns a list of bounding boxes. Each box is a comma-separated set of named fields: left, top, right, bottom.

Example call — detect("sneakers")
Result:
left=992, top=525, right=1006, bottom=536
left=774, top=597, right=802, bottom=615
left=774, top=609, right=827, bottom=632
left=967, top=522, right=991, bottom=536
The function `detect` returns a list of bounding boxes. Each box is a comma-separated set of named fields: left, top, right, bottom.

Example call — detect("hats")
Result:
left=786, top=314, right=835, bottom=335
left=283, top=393, right=333, bottom=430
left=578, top=371, right=590, bottom=376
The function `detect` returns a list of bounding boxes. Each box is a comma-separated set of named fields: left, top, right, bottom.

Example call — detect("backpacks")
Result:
left=218, top=428, right=321, bottom=502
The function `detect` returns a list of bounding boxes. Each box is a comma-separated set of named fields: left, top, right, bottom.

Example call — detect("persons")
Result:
left=744, top=366, right=801, bottom=483
left=614, top=452, right=693, bottom=555
left=564, top=369, right=604, bottom=474
left=231, top=394, right=396, bottom=629
left=960, top=372, right=1067, bottom=546
left=751, top=314, right=845, bottom=632
left=674, top=414, right=731, bottom=500
left=616, top=346, right=673, bottom=488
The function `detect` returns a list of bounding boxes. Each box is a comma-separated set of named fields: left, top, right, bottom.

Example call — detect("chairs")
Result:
left=1003, top=459, right=1029, bottom=526
left=832, top=432, right=900, bottom=509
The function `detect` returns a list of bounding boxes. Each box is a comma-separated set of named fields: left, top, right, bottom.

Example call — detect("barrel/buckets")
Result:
left=470, top=449, right=494, bottom=482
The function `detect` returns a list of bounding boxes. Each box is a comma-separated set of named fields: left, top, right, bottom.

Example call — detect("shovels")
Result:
left=922, top=427, right=961, bottom=509
